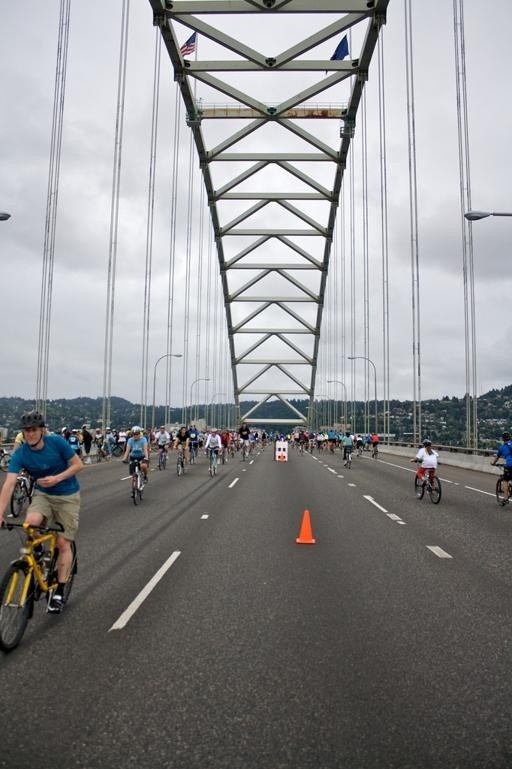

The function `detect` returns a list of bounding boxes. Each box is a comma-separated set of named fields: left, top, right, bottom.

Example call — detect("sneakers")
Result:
left=47, top=593, right=65, bottom=613
left=33, top=542, right=45, bottom=563
left=499, top=499, right=509, bottom=506
left=421, top=479, right=427, bottom=487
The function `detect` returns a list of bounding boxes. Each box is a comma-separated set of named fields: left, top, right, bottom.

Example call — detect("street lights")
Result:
left=463, top=212, right=512, bottom=222
left=0, top=212, right=10, bottom=220
left=348, top=356, right=379, bottom=436
left=210, top=392, right=227, bottom=429
left=305, top=406, right=319, bottom=430
left=228, top=405, right=240, bottom=432
left=326, top=379, right=350, bottom=433
left=190, top=378, right=209, bottom=428
left=151, top=354, right=182, bottom=428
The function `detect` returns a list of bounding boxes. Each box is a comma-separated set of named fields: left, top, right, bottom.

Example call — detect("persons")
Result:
left=411, top=439, right=439, bottom=494
left=251, top=429, right=329, bottom=451
left=0, top=413, right=84, bottom=614
left=16, top=424, right=154, bottom=497
left=490, top=431, right=512, bottom=502
left=142, top=424, right=251, bottom=468
left=328, top=429, right=379, bottom=467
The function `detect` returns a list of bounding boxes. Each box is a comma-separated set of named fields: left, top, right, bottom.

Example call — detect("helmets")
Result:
left=19, top=411, right=45, bottom=429
left=61, top=424, right=110, bottom=433
left=422, top=439, right=432, bottom=444
left=131, top=426, right=141, bottom=434
left=211, top=428, right=217, bottom=433
left=502, top=431, right=509, bottom=441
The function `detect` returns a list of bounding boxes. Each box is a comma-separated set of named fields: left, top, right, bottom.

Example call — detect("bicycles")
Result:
left=1, top=518, right=78, bottom=651
left=8, top=467, right=37, bottom=517
left=123, top=456, right=150, bottom=506
left=491, top=461, right=512, bottom=509
left=96, top=437, right=267, bottom=477
left=289, top=440, right=379, bottom=469
left=0, top=448, right=13, bottom=471
left=410, top=458, right=443, bottom=505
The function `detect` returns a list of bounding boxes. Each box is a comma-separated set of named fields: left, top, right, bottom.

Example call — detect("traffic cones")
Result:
left=295, top=509, right=315, bottom=544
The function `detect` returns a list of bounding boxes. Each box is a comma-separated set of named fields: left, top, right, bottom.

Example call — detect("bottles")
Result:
left=41, top=552, right=52, bottom=584
